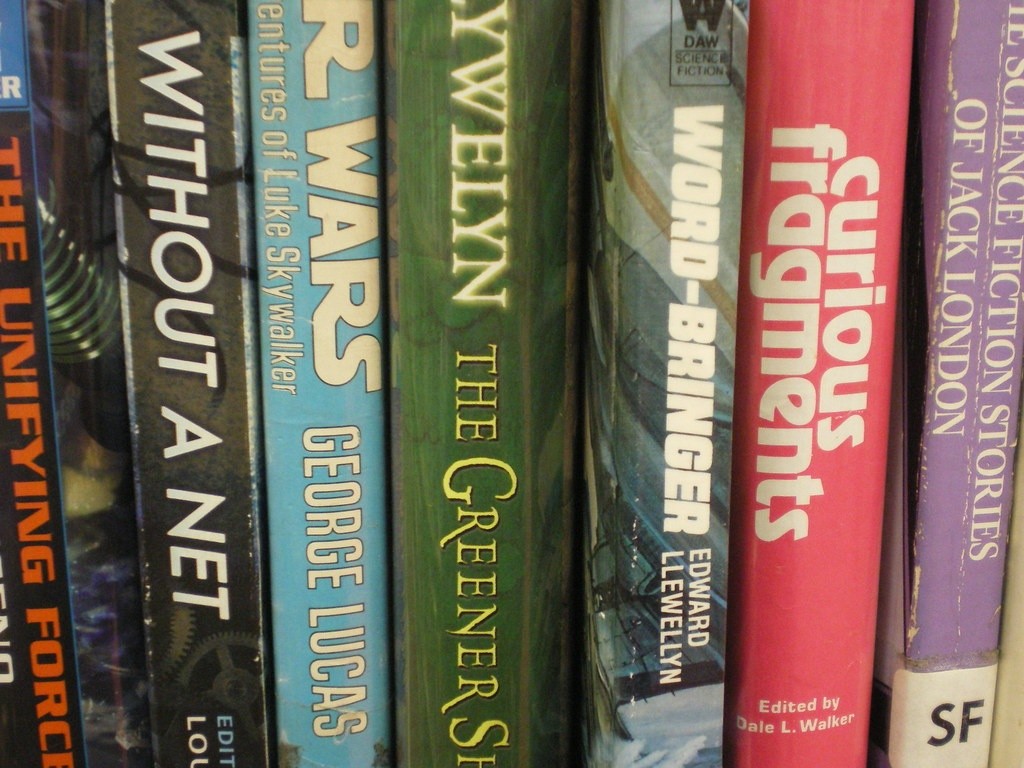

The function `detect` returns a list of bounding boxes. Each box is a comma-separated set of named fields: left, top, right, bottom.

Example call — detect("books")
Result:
left=0, top=0, right=1024, bottom=768
